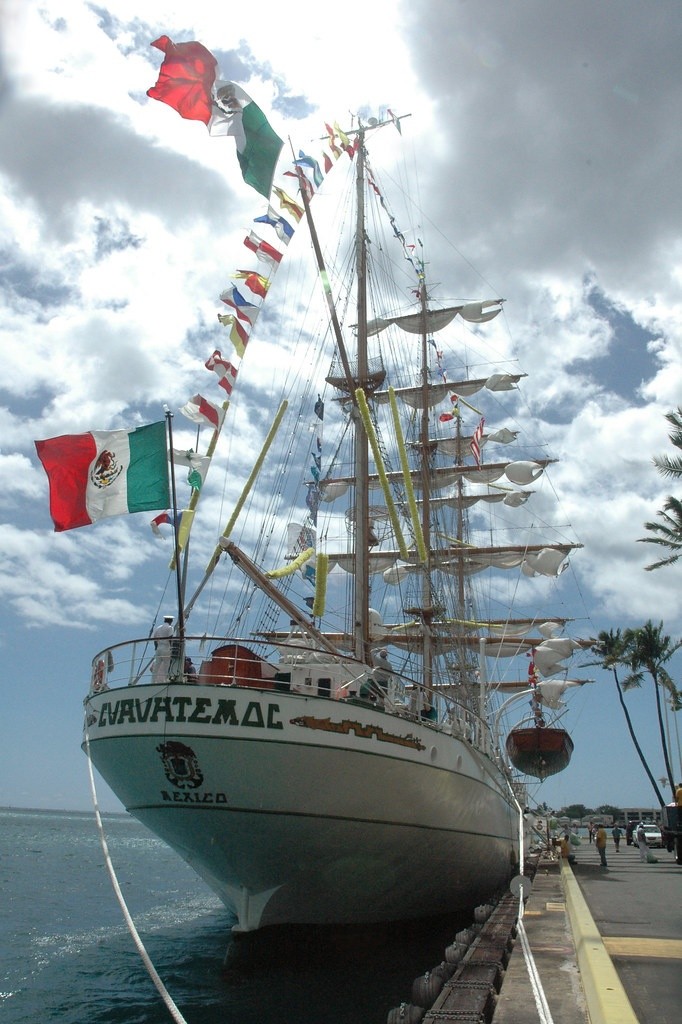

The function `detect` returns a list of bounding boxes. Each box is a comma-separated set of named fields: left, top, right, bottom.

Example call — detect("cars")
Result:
left=635, top=824, right=662, bottom=848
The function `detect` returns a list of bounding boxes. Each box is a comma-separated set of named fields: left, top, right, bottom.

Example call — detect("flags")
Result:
left=524, top=652, right=548, bottom=729
left=32, top=419, right=173, bottom=534
left=144, top=32, right=287, bottom=202
left=180, top=114, right=485, bottom=614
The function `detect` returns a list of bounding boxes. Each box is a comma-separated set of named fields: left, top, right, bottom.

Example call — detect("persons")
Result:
left=150, top=615, right=176, bottom=684
left=636, top=823, right=651, bottom=864
left=557, top=823, right=578, bottom=865
left=369, top=647, right=395, bottom=708
left=335, top=680, right=351, bottom=701
left=589, top=821, right=607, bottom=867
left=626, top=821, right=633, bottom=846
left=675, top=783, right=682, bottom=818
left=183, top=657, right=200, bottom=683
left=612, top=823, right=624, bottom=853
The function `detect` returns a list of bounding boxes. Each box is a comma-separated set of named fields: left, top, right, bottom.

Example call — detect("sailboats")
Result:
left=78, top=120, right=607, bottom=951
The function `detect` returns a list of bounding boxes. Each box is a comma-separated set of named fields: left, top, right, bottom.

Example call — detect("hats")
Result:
left=163, top=616, right=174, bottom=623
left=380, top=650, right=387, bottom=657
left=639, top=823, right=643, bottom=826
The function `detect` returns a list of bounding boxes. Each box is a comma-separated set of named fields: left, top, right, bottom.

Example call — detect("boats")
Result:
left=505, top=714, right=576, bottom=784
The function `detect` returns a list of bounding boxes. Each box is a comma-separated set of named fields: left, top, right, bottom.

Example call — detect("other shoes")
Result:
left=600, top=863, right=607, bottom=866
